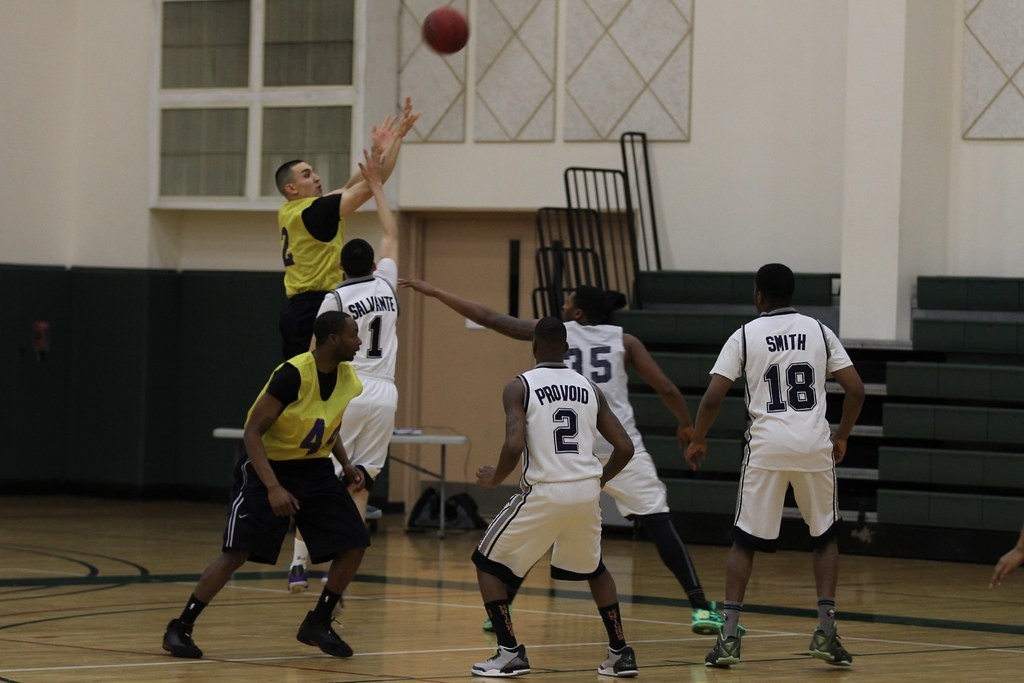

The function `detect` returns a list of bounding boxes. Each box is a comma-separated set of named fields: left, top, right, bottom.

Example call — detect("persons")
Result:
left=285, top=147, right=399, bottom=593
left=161, top=311, right=370, bottom=659
left=988, top=525, right=1024, bottom=590
left=399, top=277, right=748, bottom=635
left=276, top=95, right=421, bottom=362
left=685, top=264, right=866, bottom=666
left=471, top=318, right=638, bottom=676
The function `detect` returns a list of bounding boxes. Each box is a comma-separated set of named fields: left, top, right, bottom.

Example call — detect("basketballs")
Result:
left=422, top=6, right=470, bottom=56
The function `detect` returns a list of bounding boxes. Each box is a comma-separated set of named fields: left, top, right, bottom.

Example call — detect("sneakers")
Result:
left=162, top=618, right=203, bottom=658
left=705, top=626, right=741, bottom=666
left=470, top=643, right=532, bottom=676
left=296, top=610, right=353, bottom=656
left=809, top=622, right=853, bottom=666
left=597, top=646, right=639, bottom=677
left=289, top=561, right=310, bottom=592
left=691, top=601, right=747, bottom=636
left=320, top=577, right=345, bottom=614
left=483, top=604, right=512, bottom=630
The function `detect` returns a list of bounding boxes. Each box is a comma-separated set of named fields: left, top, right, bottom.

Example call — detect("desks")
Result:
left=212, top=428, right=467, bottom=541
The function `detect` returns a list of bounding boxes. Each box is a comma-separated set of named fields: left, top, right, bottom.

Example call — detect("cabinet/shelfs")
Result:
left=148, top=0, right=399, bottom=212
